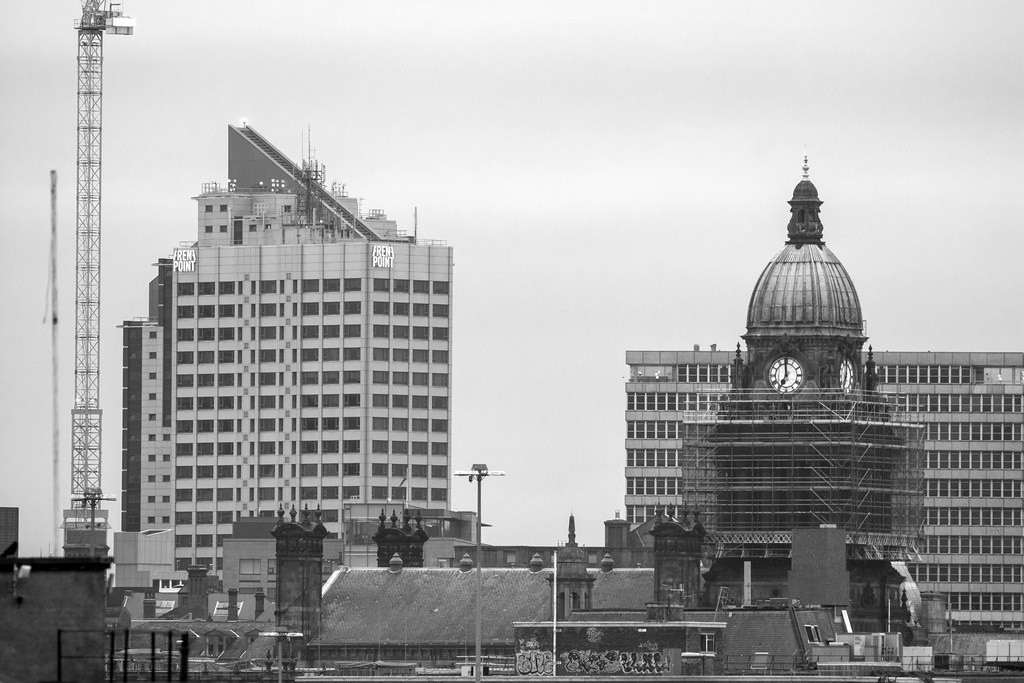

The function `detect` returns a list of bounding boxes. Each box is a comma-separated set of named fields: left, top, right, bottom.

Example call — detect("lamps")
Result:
left=12, top=563, right=32, bottom=599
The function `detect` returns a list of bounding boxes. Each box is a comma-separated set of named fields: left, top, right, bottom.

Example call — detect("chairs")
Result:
left=743, top=650, right=776, bottom=675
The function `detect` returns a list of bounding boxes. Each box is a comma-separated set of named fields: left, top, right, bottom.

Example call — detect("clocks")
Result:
left=768, top=356, right=804, bottom=393
left=839, top=356, right=855, bottom=397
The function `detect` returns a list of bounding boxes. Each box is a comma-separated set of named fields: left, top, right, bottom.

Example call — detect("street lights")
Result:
left=452, top=464, right=509, bottom=681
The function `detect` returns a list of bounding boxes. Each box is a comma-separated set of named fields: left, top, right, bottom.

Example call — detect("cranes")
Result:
left=64, top=0, right=137, bottom=514
